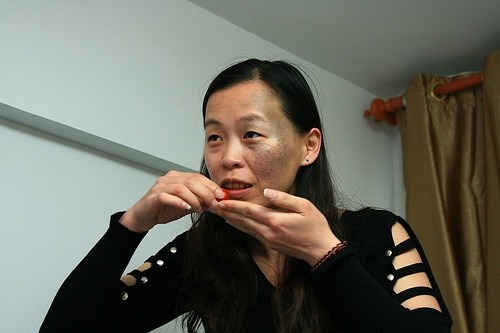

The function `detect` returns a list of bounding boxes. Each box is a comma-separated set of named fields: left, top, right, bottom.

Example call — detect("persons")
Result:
left=37, top=56, right=455, bottom=333
